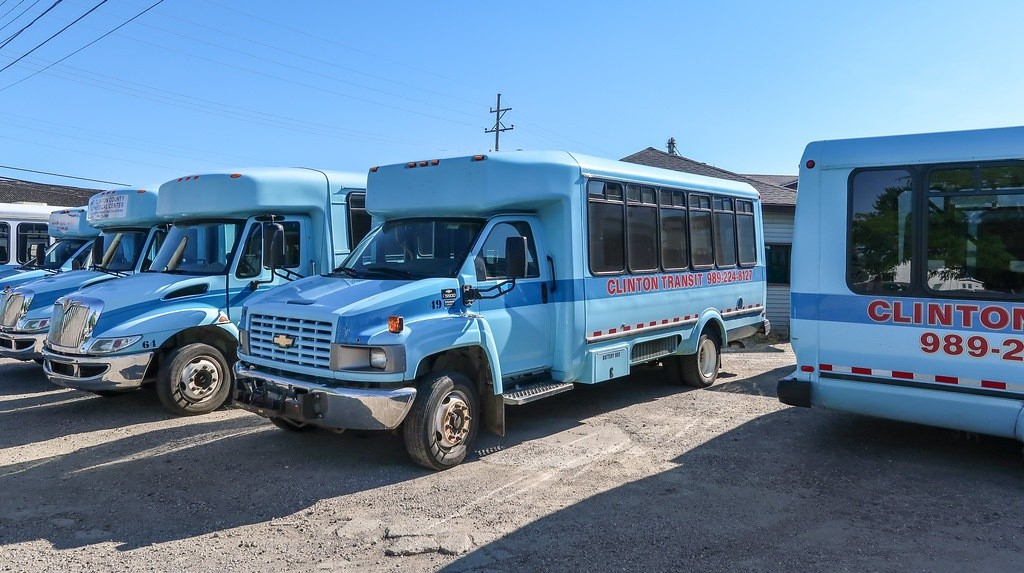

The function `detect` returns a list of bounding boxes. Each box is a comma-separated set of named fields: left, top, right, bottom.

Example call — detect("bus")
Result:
left=777, top=126, right=1023, bottom=442
left=232, top=149, right=770, bottom=471
left=0, top=187, right=174, bottom=367
left=41, top=167, right=473, bottom=414
